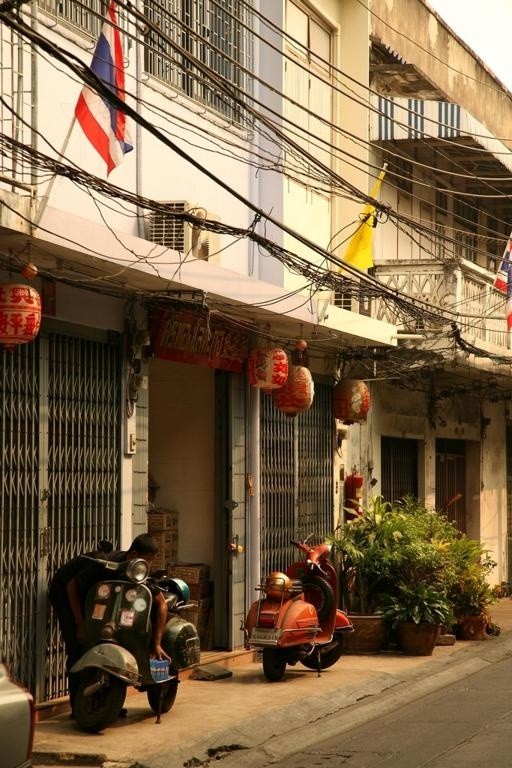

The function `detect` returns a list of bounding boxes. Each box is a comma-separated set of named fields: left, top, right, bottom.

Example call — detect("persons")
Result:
left=46, top=533, right=172, bottom=719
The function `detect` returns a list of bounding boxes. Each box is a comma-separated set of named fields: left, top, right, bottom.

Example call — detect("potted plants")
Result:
left=325, top=493, right=501, bottom=656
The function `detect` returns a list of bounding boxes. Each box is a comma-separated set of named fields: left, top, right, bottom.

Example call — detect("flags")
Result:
left=74, top=3, right=134, bottom=181
left=491, top=230, right=512, bottom=334
left=339, top=168, right=386, bottom=278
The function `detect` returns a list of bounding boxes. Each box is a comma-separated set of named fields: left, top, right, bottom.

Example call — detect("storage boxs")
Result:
left=151, top=512, right=210, bottom=650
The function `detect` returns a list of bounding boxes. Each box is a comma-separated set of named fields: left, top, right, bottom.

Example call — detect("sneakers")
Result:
left=118, top=709, right=128, bottom=717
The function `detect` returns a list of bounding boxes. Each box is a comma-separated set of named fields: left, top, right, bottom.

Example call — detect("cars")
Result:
left=0, top=660, right=38, bottom=767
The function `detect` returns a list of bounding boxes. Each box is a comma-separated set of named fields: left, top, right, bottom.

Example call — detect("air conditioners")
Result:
left=333, top=273, right=371, bottom=317
left=147, top=200, right=221, bottom=265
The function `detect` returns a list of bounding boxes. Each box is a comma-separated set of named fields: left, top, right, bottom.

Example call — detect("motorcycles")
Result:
left=241, top=530, right=356, bottom=681
left=62, top=542, right=199, bottom=732
